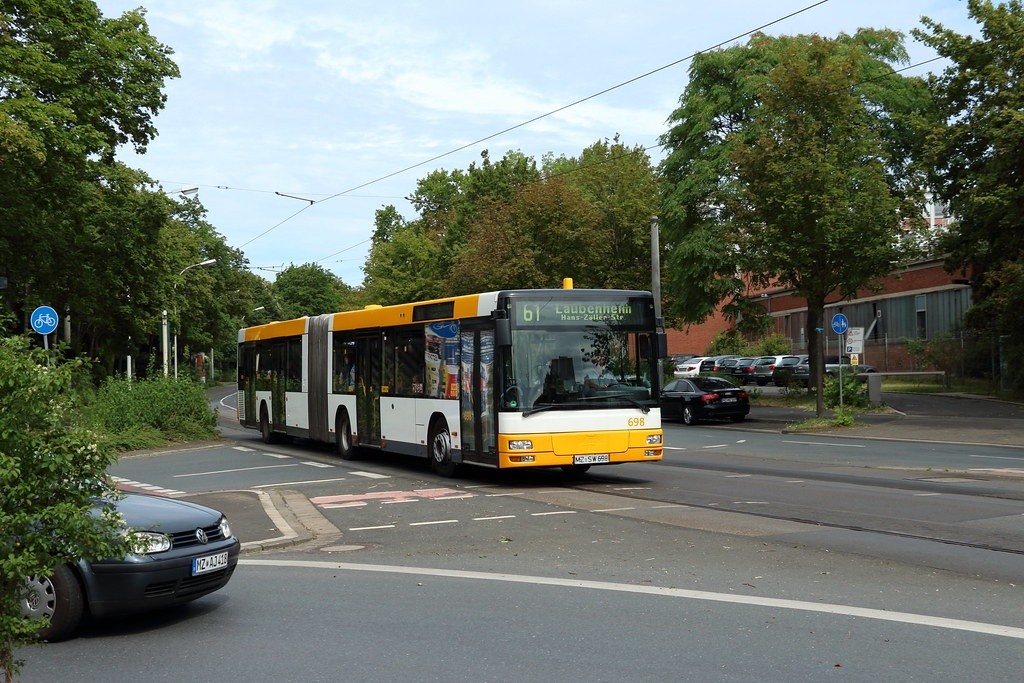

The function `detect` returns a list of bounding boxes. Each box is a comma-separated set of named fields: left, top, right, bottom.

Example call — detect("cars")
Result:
left=773, top=354, right=878, bottom=389
left=661, top=376, right=750, bottom=426
left=671, top=354, right=743, bottom=378
left=9, top=480, right=241, bottom=643
left=753, top=355, right=809, bottom=386
left=733, top=356, right=760, bottom=383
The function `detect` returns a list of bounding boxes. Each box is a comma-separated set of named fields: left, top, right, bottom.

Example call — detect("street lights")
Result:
left=174, top=259, right=217, bottom=382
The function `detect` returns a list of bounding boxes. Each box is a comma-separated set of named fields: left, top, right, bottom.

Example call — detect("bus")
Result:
left=235, top=277, right=668, bottom=476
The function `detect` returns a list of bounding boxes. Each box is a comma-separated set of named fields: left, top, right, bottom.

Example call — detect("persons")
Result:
left=583, top=355, right=619, bottom=402
